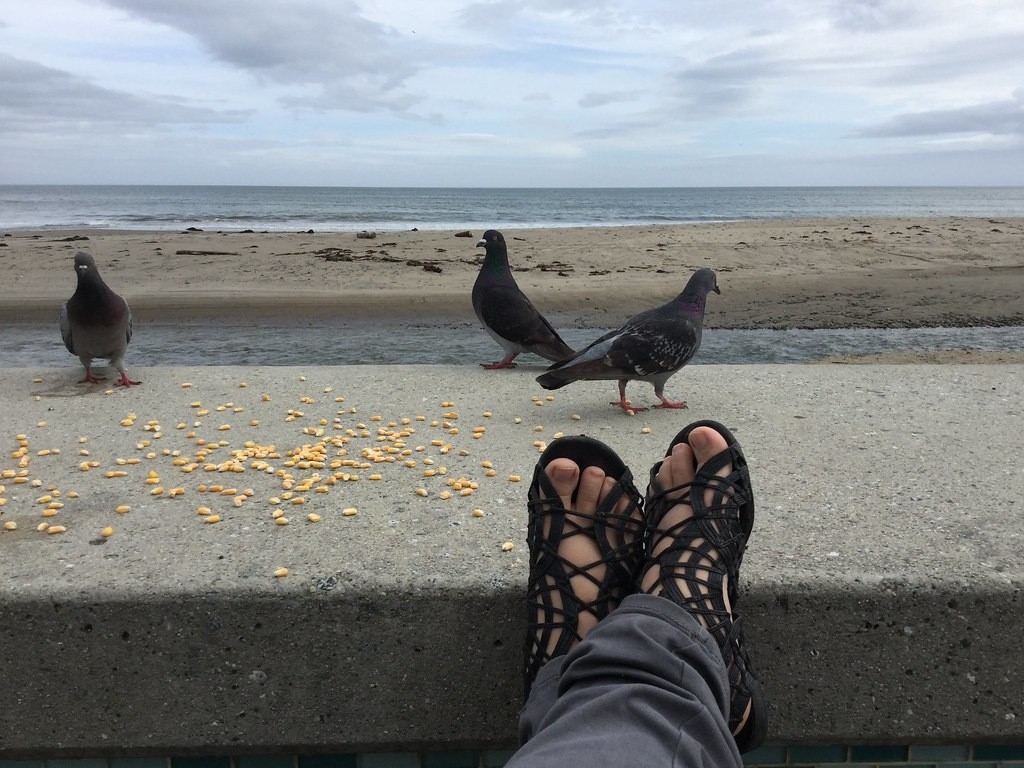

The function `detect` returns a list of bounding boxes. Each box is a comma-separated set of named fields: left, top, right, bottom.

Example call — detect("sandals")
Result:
left=522, top=434, right=644, bottom=709
left=635, top=419, right=767, bottom=755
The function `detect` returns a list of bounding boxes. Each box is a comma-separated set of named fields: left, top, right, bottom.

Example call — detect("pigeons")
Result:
left=535, top=266, right=721, bottom=416
left=471, top=229, right=576, bottom=371
left=59, top=251, right=143, bottom=388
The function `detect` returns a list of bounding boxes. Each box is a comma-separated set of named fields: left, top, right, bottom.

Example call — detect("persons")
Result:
left=504, top=419, right=769, bottom=768
